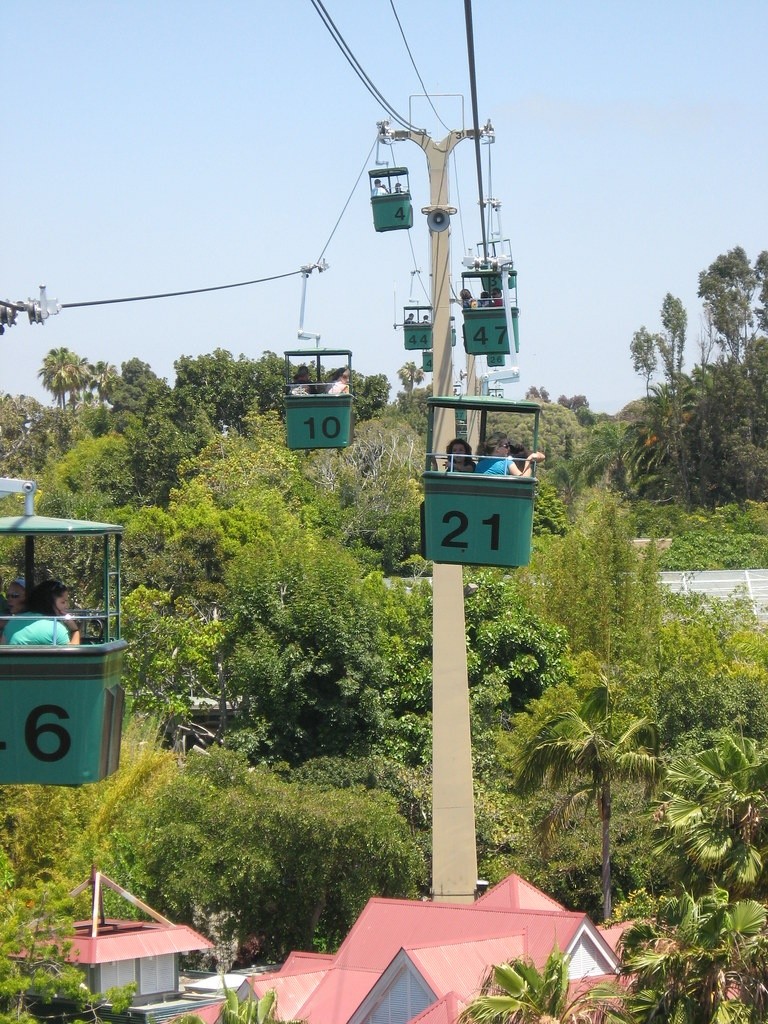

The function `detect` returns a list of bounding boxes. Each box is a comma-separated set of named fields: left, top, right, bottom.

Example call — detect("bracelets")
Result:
left=71, top=629, right=79, bottom=635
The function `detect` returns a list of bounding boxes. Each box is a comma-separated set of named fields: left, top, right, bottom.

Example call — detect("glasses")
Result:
left=6, top=594, right=20, bottom=599
left=499, top=443, right=509, bottom=449
left=51, top=582, right=63, bottom=592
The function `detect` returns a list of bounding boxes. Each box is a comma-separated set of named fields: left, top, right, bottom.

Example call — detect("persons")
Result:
left=404, top=313, right=417, bottom=324
left=443, top=439, right=476, bottom=473
left=476, top=287, right=503, bottom=307
left=476, top=431, right=546, bottom=477
left=394, top=183, right=405, bottom=193
left=288, top=366, right=318, bottom=395
left=2, top=580, right=81, bottom=646
left=0, top=577, right=34, bottom=633
left=322, top=367, right=351, bottom=394
left=371, top=179, right=389, bottom=197
left=460, top=289, right=482, bottom=308
left=421, top=315, right=430, bottom=324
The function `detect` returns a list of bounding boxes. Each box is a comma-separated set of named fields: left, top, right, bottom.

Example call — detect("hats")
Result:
left=15, top=577, right=25, bottom=588
